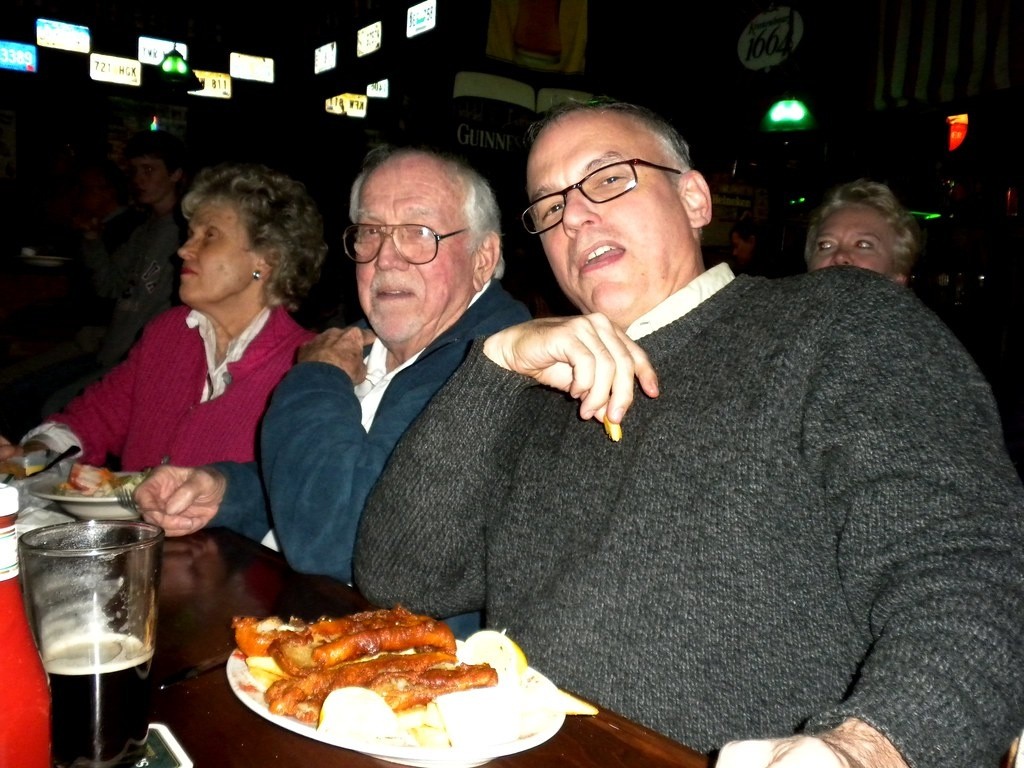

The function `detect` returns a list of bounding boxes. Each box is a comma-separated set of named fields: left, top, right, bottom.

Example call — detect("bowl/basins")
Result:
left=28, top=472, right=144, bottom=522
left=0, top=455, right=72, bottom=510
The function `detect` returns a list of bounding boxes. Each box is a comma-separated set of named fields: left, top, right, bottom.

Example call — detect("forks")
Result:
left=115, top=488, right=139, bottom=516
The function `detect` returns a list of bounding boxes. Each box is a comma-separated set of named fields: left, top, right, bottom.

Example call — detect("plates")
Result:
left=227, top=647, right=566, bottom=768
left=17, top=254, right=71, bottom=266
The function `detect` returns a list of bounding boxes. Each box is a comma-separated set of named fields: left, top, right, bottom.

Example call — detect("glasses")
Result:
left=344, top=223, right=471, bottom=264
left=522, top=158, right=683, bottom=234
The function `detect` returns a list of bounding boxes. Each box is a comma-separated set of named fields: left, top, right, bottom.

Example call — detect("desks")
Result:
left=43, top=501, right=717, bottom=768
left=0, top=254, right=96, bottom=392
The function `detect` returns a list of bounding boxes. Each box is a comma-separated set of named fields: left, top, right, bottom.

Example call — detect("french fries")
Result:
left=605, top=414, right=622, bottom=441
left=245, top=648, right=451, bottom=747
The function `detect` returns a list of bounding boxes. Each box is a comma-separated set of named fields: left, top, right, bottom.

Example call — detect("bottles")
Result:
left=1, top=481, right=53, bottom=767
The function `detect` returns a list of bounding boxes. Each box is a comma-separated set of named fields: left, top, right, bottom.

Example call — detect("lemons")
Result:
left=316, top=686, right=417, bottom=746
left=458, top=630, right=529, bottom=675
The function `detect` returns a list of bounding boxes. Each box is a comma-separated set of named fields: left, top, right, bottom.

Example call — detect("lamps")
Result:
left=759, top=0, right=818, bottom=132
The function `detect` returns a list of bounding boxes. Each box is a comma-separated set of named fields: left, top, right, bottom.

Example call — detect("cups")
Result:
left=17, top=520, right=165, bottom=768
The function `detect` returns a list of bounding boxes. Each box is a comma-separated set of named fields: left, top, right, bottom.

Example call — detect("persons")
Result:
left=41, top=130, right=185, bottom=419
left=350, top=98, right=1024, bottom=768
left=134, top=142, right=531, bottom=640
left=0, top=158, right=322, bottom=471
left=5, top=155, right=144, bottom=342
left=728, top=217, right=766, bottom=265
left=804, top=177, right=923, bottom=290
